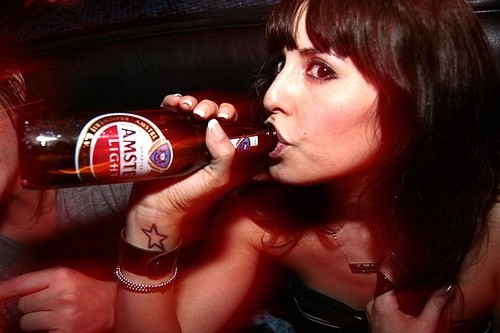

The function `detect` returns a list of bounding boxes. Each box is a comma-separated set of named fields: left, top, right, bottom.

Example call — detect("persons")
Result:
left=0, top=56, right=132, bottom=333
left=114, top=0, right=500, bottom=333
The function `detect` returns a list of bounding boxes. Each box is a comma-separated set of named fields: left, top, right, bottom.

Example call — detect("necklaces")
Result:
left=323, top=219, right=403, bottom=273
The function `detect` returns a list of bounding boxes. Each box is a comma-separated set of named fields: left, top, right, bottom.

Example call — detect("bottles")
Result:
left=18, top=107, right=277, bottom=187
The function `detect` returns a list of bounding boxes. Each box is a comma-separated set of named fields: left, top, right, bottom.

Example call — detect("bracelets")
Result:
left=119, top=227, right=183, bottom=278
left=114, top=263, right=178, bottom=293
